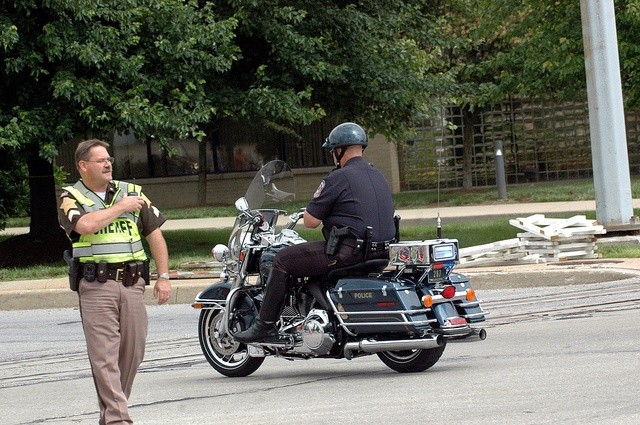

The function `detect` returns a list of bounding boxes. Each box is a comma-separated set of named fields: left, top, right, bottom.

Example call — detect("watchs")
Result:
left=156, top=272, right=170, bottom=280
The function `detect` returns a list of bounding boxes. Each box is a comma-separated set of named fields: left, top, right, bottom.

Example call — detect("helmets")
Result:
left=322, top=122, right=368, bottom=148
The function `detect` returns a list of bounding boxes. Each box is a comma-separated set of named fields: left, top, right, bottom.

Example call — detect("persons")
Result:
left=55, top=136, right=173, bottom=425
left=231, top=121, right=402, bottom=344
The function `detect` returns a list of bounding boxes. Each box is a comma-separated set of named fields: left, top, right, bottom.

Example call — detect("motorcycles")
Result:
left=191, top=160, right=490, bottom=376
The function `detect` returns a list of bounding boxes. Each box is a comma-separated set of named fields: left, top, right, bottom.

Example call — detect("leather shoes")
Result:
left=233, top=321, right=279, bottom=343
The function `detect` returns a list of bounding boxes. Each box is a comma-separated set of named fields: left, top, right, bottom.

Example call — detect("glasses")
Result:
left=84, top=157, right=115, bottom=165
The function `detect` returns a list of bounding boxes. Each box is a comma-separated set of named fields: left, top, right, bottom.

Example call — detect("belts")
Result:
left=342, top=237, right=397, bottom=252
left=79, top=264, right=147, bottom=282
